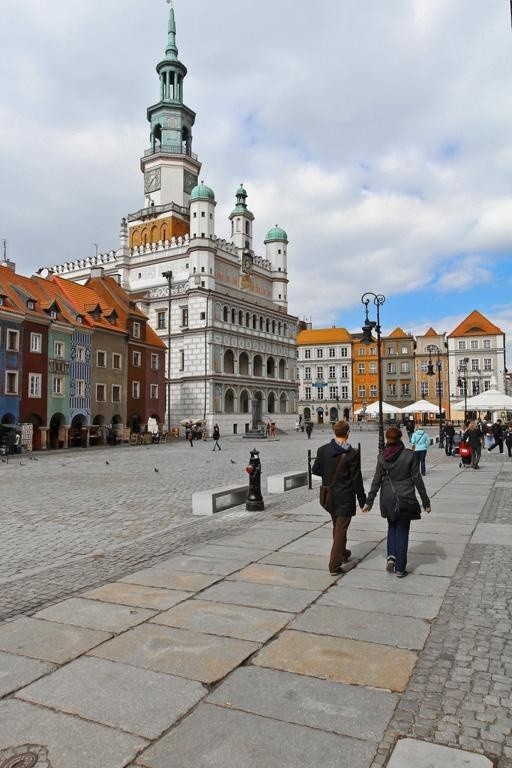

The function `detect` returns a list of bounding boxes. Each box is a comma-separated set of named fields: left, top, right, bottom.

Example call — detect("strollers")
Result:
left=458, top=439, right=472, bottom=469
left=451, top=432, right=463, bottom=456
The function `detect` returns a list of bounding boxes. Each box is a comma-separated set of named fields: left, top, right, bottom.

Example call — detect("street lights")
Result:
left=455, top=364, right=468, bottom=422
left=358, top=290, right=387, bottom=456
left=422, top=344, right=446, bottom=448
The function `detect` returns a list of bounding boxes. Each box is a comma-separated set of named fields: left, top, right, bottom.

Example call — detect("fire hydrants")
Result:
left=243, top=448, right=266, bottom=512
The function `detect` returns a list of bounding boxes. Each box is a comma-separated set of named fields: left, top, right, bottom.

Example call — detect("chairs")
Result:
left=131, top=431, right=176, bottom=445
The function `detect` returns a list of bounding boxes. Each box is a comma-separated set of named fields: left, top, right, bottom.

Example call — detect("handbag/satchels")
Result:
left=394, top=495, right=421, bottom=516
left=320, top=486, right=333, bottom=513
left=412, top=444, right=415, bottom=451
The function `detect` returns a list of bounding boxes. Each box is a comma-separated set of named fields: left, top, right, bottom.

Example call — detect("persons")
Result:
left=364, top=427, right=432, bottom=578
left=295, top=417, right=315, bottom=439
left=437, top=415, right=512, bottom=469
left=213, top=423, right=221, bottom=451
left=312, top=420, right=367, bottom=576
left=410, top=424, right=431, bottom=475
left=405, top=419, right=415, bottom=442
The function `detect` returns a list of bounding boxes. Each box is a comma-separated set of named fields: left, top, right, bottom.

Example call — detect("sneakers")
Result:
left=396, top=571, right=408, bottom=578
left=341, top=550, right=351, bottom=563
left=330, top=566, right=343, bottom=577
left=386, top=557, right=395, bottom=572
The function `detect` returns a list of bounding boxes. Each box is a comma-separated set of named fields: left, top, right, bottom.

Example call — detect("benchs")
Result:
left=192, top=483, right=249, bottom=516
left=429, top=436, right=439, bottom=445
left=266, top=471, right=308, bottom=494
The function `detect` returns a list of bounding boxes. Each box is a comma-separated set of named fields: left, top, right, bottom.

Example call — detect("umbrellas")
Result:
left=400, top=399, right=448, bottom=413
left=352, top=400, right=401, bottom=415
left=452, top=387, right=512, bottom=410
